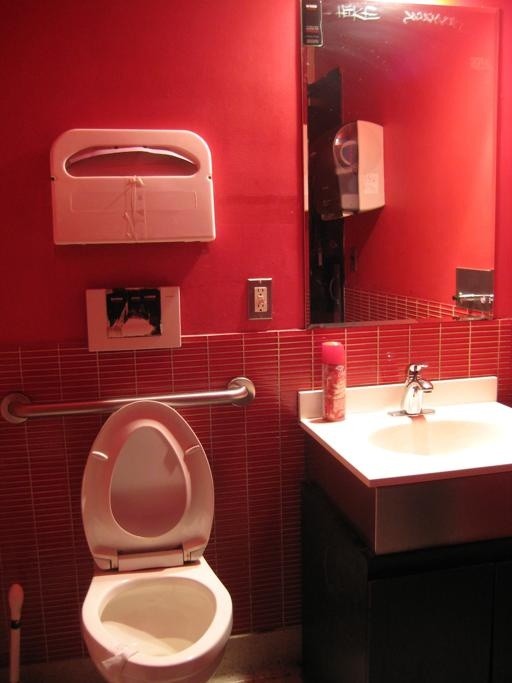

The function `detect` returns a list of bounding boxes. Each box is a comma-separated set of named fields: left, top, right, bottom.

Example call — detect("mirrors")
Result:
left=302, top=0, right=501, bottom=330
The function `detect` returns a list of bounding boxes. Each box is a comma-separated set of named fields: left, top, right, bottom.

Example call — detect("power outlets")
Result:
left=247, top=278, right=272, bottom=320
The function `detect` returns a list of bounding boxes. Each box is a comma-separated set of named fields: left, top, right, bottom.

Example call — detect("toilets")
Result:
left=78, top=399, right=235, bottom=683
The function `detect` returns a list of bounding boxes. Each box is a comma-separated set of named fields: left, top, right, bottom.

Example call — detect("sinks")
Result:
left=369, top=419, right=512, bottom=457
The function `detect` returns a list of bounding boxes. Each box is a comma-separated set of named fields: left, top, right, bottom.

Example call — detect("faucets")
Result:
left=399, top=363, right=433, bottom=415
